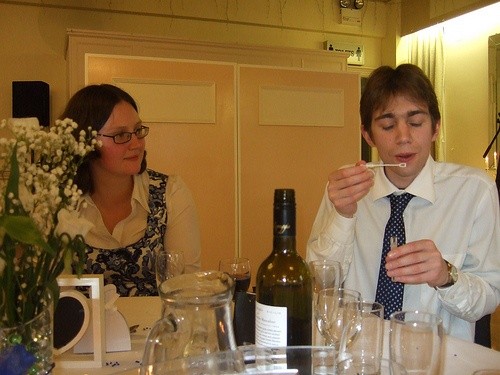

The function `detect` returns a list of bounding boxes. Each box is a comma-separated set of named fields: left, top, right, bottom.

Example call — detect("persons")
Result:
left=306, top=64, right=500, bottom=341
left=15, top=84, right=199, bottom=297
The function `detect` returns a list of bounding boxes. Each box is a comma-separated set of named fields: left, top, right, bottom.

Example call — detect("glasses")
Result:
left=97, top=126, right=150, bottom=144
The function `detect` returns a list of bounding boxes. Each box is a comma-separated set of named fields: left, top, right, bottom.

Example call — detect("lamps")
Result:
left=339, top=0, right=364, bottom=26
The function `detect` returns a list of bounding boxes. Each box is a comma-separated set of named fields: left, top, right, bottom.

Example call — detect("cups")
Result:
left=155, top=249, right=185, bottom=283
left=340, top=301, right=384, bottom=375
left=386, top=309, right=445, bottom=374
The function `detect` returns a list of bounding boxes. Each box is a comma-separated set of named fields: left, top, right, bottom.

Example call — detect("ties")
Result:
left=371, top=192, right=416, bottom=323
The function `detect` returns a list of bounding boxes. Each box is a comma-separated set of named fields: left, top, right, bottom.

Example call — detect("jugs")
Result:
left=138, top=270, right=245, bottom=375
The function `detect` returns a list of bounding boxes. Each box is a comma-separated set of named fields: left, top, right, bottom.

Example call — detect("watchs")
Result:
left=434, top=259, right=458, bottom=291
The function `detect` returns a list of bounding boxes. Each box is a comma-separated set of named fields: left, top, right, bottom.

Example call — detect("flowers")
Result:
left=0, top=119, right=103, bottom=354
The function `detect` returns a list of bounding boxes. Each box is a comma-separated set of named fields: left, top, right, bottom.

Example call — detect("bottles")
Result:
left=256, top=188, right=312, bottom=375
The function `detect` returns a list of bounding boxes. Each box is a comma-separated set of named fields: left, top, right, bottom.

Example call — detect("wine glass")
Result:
left=219, top=256, right=251, bottom=292
left=308, top=260, right=363, bottom=375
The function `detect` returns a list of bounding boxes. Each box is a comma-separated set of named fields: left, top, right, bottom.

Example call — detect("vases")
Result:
left=0, top=302, right=51, bottom=375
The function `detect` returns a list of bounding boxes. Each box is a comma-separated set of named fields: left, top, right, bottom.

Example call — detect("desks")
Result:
left=51, top=295, right=500, bottom=375
